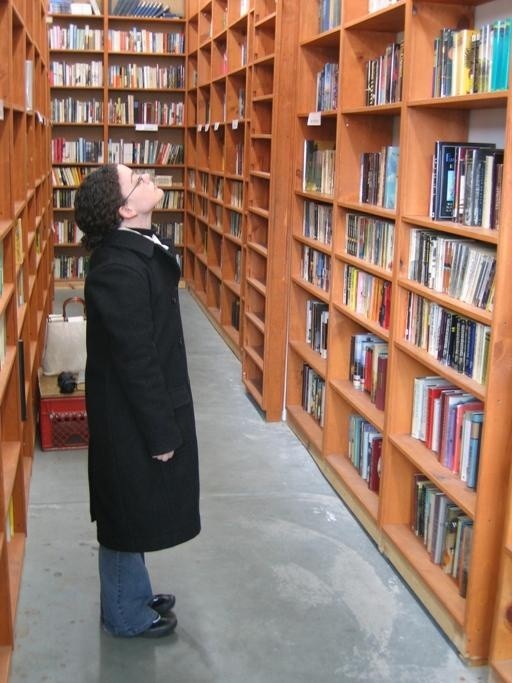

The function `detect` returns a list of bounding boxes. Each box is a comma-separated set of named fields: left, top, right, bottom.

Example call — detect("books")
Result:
left=188, top=169, right=224, bottom=227
left=342, top=263, right=392, bottom=329
left=241, top=41, right=247, bottom=66
left=359, top=145, right=399, bottom=210
left=347, top=412, right=385, bottom=491
left=365, top=41, right=403, bottom=106
left=411, top=375, right=485, bottom=490
left=429, top=140, right=504, bottom=230
left=235, top=251, right=241, bottom=284
left=230, top=211, right=242, bottom=237
left=236, top=144, right=244, bottom=175
left=231, top=298, right=240, bottom=331
left=432, top=18, right=512, bottom=97
left=410, top=473, right=474, bottom=598
left=408, top=229, right=498, bottom=311
left=238, top=88, right=245, bottom=121
left=404, top=290, right=491, bottom=385
left=316, top=62, right=338, bottom=112
left=231, top=182, right=243, bottom=208
left=45, top=10, right=188, bottom=274
left=320, top=0, right=341, bottom=32
left=301, top=139, right=335, bottom=429
left=349, top=332, right=389, bottom=411
left=345, top=212, right=396, bottom=272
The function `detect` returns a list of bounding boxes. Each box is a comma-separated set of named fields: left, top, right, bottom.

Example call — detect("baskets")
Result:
left=39, top=394, right=88, bottom=453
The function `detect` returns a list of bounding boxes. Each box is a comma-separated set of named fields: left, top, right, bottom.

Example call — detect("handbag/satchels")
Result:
left=41, top=295, right=88, bottom=378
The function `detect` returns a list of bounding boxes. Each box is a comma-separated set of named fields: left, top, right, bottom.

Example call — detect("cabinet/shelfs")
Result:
left=178, top=0, right=512, bottom=680
left=50, top=6, right=184, bottom=286
left=0, top=2, right=51, bottom=683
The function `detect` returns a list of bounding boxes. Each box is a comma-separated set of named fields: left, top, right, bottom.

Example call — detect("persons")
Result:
left=73, top=160, right=201, bottom=639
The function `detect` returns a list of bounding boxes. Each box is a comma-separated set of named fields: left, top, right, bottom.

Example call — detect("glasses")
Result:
left=116, top=174, right=143, bottom=212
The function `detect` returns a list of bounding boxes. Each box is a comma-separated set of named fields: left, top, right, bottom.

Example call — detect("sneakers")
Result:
left=140, top=592, right=177, bottom=639
